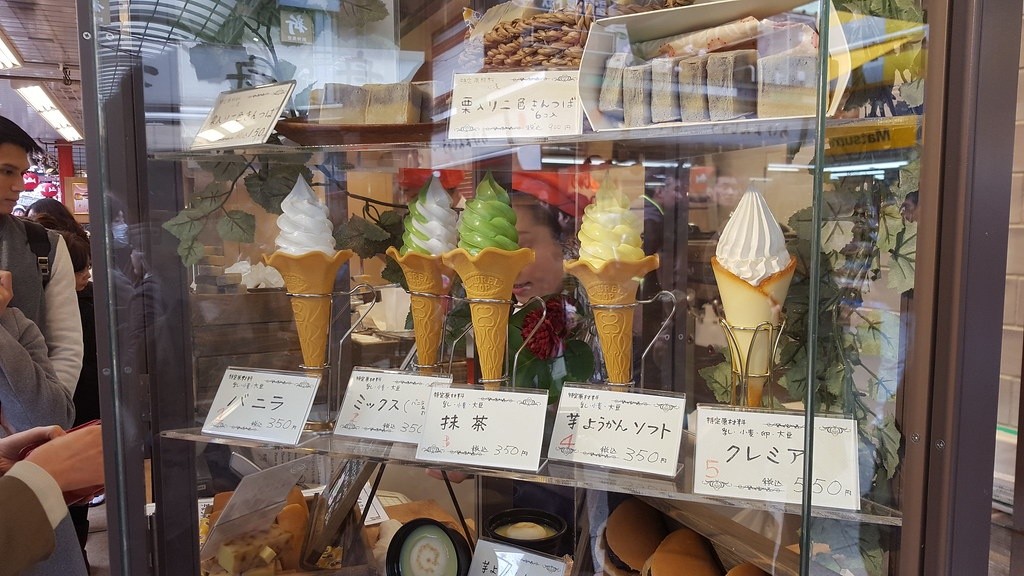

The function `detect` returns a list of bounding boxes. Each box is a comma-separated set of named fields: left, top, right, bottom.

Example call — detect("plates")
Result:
left=274, top=117, right=450, bottom=146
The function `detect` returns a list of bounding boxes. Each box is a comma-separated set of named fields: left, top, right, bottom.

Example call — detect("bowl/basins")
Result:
left=484, top=508, right=567, bottom=557
left=385, top=518, right=472, bottom=576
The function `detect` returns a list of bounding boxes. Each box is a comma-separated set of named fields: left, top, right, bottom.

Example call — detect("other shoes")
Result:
left=89, top=495, right=104, bottom=508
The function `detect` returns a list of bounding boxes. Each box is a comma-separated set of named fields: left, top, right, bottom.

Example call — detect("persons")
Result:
left=633, top=170, right=682, bottom=351
left=0, top=114, right=179, bottom=575
left=396, top=166, right=603, bottom=557
left=900, top=190, right=918, bottom=223
left=704, top=169, right=739, bottom=206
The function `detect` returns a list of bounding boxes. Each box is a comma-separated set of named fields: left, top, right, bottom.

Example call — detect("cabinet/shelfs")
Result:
left=73, top=0, right=996, bottom=576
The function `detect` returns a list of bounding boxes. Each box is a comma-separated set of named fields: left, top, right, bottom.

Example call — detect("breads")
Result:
left=200, top=482, right=315, bottom=576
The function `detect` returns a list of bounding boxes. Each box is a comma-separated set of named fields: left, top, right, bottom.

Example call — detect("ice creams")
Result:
left=710, top=179, right=798, bottom=408
left=383, top=175, right=461, bottom=376
left=440, top=169, right=536, bottom=390
left=563, top=168, right=660, bottom=391
left=262, top=173, right=354, bottom=382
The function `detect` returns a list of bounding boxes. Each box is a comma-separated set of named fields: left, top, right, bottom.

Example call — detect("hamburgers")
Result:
left=591, top=496, right=772, bottom=576
